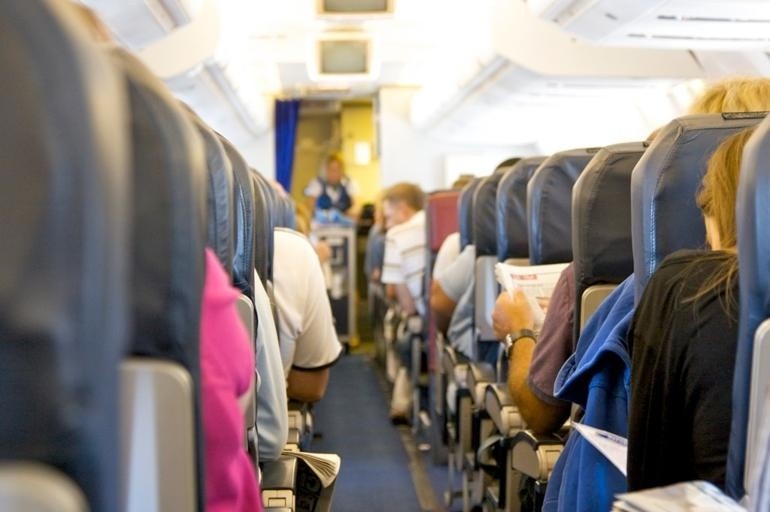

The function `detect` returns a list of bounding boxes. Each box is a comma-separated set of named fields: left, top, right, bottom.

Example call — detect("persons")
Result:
left=488, top=259, right=577, bottom=437
left=302, top=153, right=354, bottom=352
left=265, top=224, right=346, bottom=410
left=531, top=70, right=770, bottom=510
left=355, top=158, right=518, bottom=446
left=618, top=125, right=754, bottom=494
left=201, top=246, right=270, bottom=512
left=245, top=270, right=291, bottom=460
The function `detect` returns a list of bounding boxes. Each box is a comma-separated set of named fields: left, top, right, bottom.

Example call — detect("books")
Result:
left=283, top=449, right=342, bottom=512
left=608, top=478, right=754, bottom=512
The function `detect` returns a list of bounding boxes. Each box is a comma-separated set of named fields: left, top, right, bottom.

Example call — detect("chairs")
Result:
left=362, top=112, right=770, bottom=512
left=0, top=2, right=311, bottom=511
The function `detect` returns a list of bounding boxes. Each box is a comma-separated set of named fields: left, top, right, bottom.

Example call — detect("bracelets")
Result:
left=501, top=328, right=538, bottom=360
